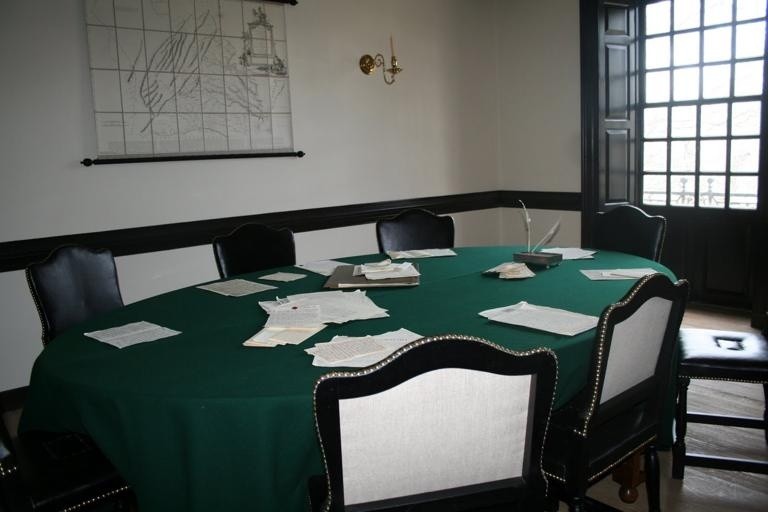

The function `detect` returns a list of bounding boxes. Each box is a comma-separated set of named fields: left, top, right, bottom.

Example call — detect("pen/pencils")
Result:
left=610, top=273, right=640, bottom=279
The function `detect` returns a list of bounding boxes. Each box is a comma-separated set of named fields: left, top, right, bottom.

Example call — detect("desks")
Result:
left=28, top=242, right=681, bottom=509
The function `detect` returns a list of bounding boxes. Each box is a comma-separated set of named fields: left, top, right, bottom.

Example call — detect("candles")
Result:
left=390, top=34, right=395, bottom=57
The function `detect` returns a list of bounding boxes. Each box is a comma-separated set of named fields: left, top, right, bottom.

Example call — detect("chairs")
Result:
left=540, top=273, right=694, bottom=511
left=586, top=202, right=668, bottom=263
left=306, top=334, right=559, bottom=511
left=22, top=244, right=127, bottom=346
left=670, top=308, right=767, bottom=482
left=372, top=208, right=456, bottom=252
left=212, top=223, right=296, bottom=282
left=0, top=386, right=135, bottom=512
